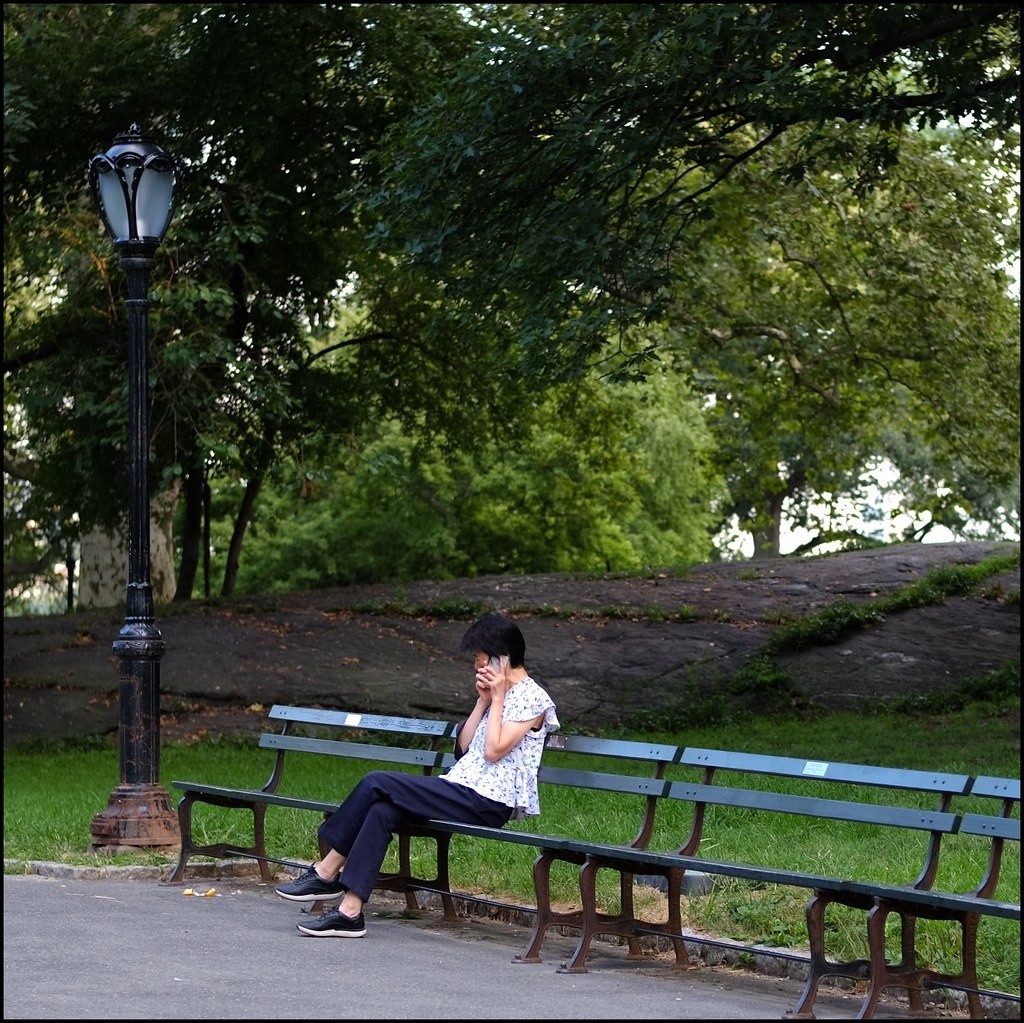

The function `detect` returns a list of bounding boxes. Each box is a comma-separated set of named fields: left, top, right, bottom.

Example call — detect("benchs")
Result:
left=167, top=705, right=1020, bottom=1019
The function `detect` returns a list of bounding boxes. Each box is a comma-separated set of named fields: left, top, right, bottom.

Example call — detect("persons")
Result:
left=275, top=615, right=560, bottom=938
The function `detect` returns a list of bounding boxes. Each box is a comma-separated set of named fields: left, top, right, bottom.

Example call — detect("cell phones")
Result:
left=485, top=655, right=509, bottom=689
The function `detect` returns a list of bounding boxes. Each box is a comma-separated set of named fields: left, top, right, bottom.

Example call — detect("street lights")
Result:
left=77, top=118, right=178, bottom=852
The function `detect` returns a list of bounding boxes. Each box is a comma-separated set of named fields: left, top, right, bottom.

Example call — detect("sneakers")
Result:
left=274, top=861, right=344, bottom=902
left=297, top=905, right=366, bottom=938
